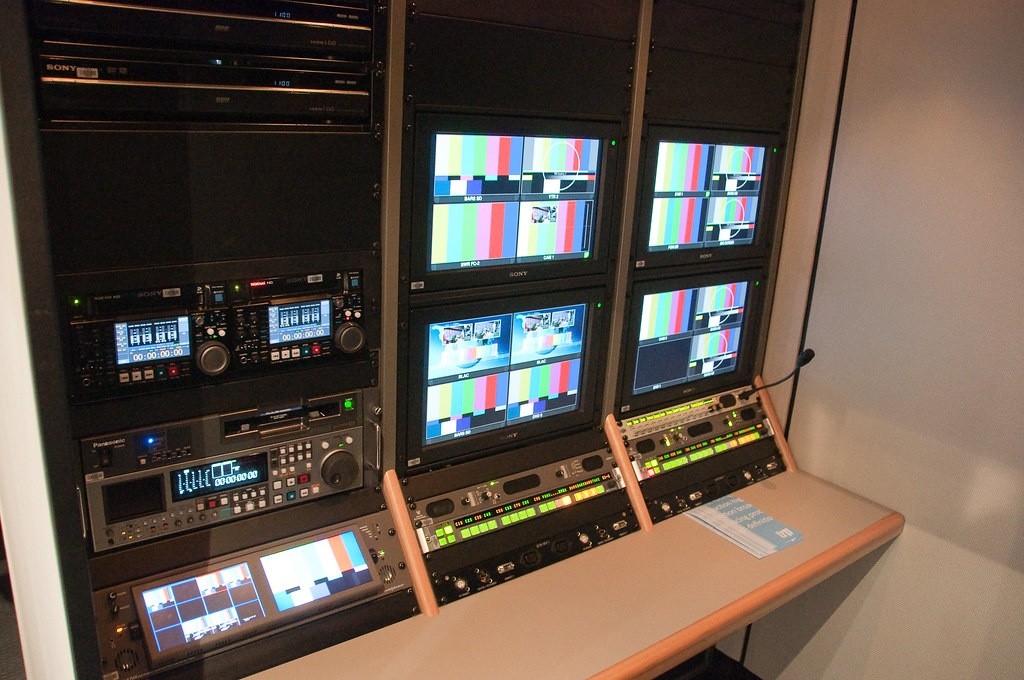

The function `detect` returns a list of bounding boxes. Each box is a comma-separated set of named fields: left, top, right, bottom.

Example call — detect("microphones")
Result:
left=737, top=348, right=814, bottom=399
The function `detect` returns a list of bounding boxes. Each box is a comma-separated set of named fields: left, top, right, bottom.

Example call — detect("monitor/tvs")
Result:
left=636, top=119, right=784, bottom=268
left=619, top=268, right=762, bottom=414
left=406, top=289, right=604, bottom=469
left=410, top=101, right=618, bottom=289
